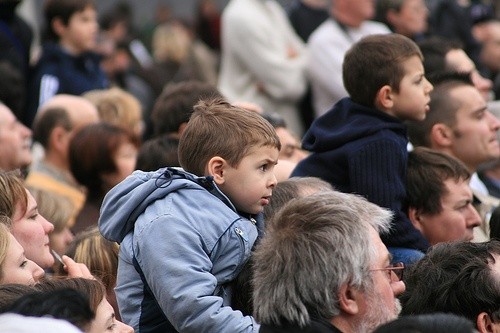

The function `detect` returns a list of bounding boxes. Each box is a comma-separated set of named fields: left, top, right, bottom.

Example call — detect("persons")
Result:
left=0, top=104, right=33, bottom=169
left=264, top=113, right=308, bottom=184
left=249, top=191, right=404, bottom=333
left=25, top=184, right=73, bottom=253
left=74, top=230, right=121, bottom=321
left=56, top=278, right=135, bottom=333
left=85, top=88, right=146, bottom=137
left=377, top=314, right=474, bottom=333
left=98, top=98, right=282, bottom=333
left=0, top=1, right=35, bottom=124
left=65, top=124, right=136, bottom=233
left=152, top=84, right=226, bottom=134
left=0, top=290, right=96, bottom=333
left=398, top=241, right=500, bottom=333
left=405, top=147, right=481, bottom=245
left=289, top=34, right=434, bottom=266
left=416, top=81, right=500, bottom=245
left=217, top=1, right=305, bottom=142
left=0, top=284, right=49, bottom=311
left=0, top=220, right=44, bottom=284
left=135, top=136, right=181, bottom=172
left=27, top=94, right=103, bottom=229
left=0, top=0, right=500, bottom=116
left=0, top=170, right=97, bottom=281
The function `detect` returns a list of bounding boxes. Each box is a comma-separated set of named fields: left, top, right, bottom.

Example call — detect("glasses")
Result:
left=367, top=260, right=405, bottom=284
